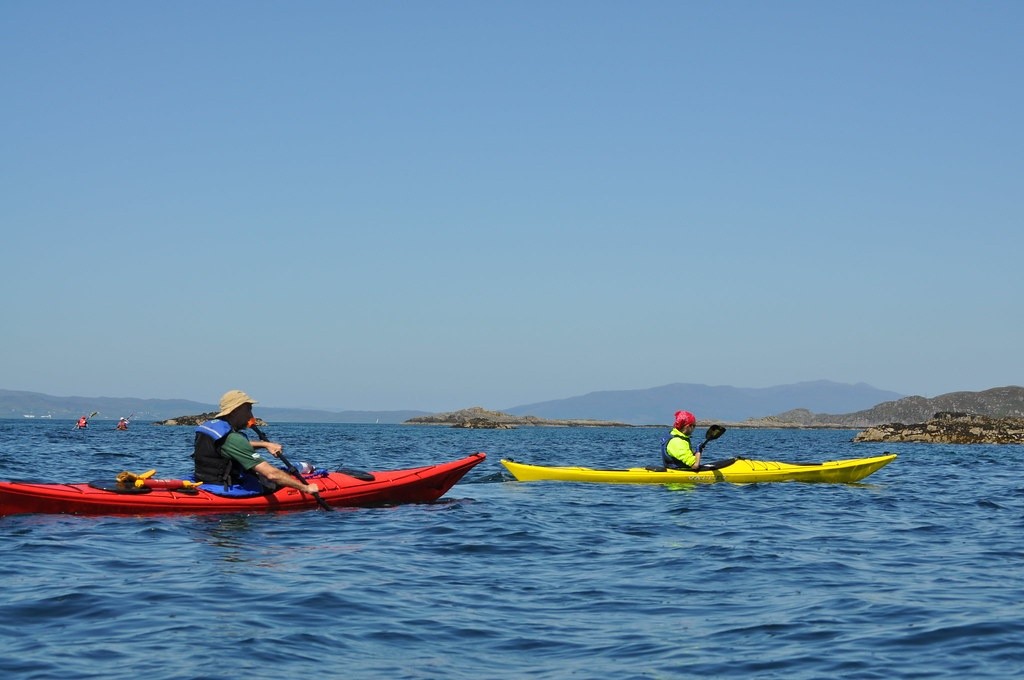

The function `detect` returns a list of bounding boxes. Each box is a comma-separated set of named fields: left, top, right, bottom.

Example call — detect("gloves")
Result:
left=695, top=442, right=706, bottom=452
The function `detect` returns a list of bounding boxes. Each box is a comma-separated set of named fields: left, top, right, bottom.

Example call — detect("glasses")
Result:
left=690, top=424, right=696, bottom=428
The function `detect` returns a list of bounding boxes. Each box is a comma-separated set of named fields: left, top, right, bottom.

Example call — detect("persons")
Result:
left=661, top=409, right=705, bottom=470
left=191, top=390, right=319, bottom=496
left=76, top=416, right=89, bottom=430
left=117, top=417, right=130, bottom=430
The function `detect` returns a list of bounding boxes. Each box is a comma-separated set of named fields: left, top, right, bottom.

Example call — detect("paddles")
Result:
left=71, top=411, right=98, bottom=431
left=693, top=424, right=727, bottom=456
left=116, top=413, right=133, bottom=429
left=247, top=414, right=330, bottom=509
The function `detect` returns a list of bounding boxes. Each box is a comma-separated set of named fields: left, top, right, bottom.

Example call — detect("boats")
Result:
left=1, top=452, right=487, bottom=515
left=499, top=453, right=898, bottom=483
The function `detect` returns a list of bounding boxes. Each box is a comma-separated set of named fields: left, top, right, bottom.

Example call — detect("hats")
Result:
left=214, top=390, right=259, bottom=418
left=674, top=411, right=694, bottom=430
left=82, top=416, right=86, bottom=419
left=120, top=417, right=124, bottom=421
